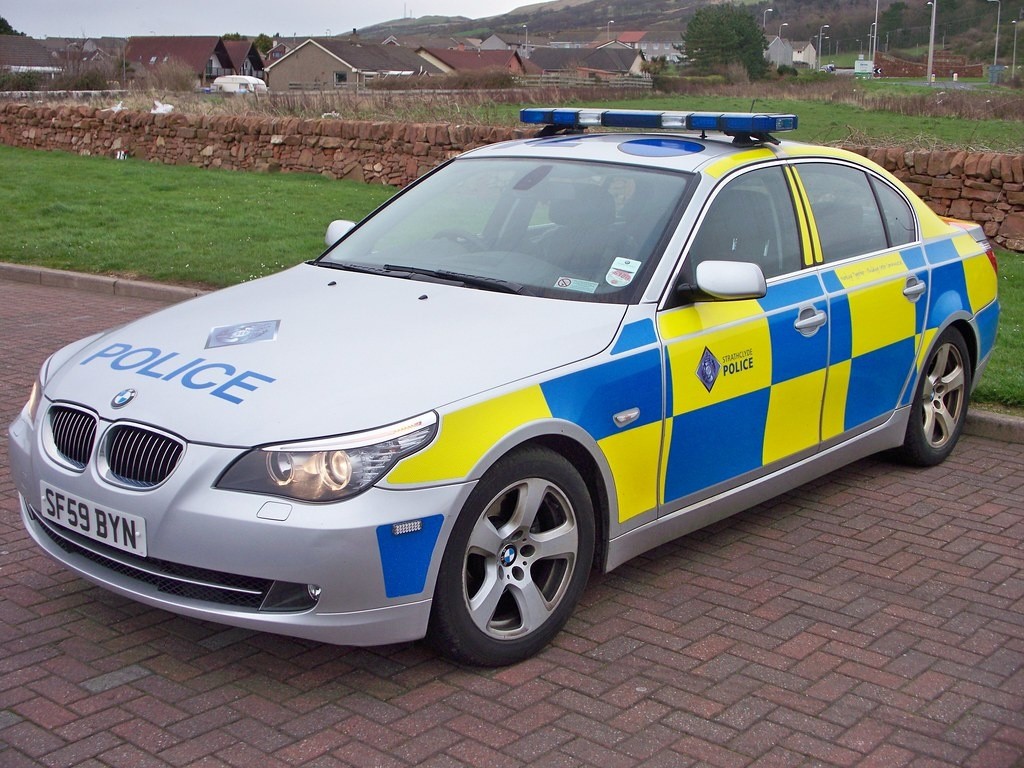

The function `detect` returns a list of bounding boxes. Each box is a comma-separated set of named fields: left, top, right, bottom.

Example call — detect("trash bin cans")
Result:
left=931, top=74, right=935, bottom=82
left=953, top=73, right=958, bottom=82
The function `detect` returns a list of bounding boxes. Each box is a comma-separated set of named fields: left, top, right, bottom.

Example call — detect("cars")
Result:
left=641, top=52, right=697, bottom=63
left=7, top=102, right=1004, bottom=664
left=820, top=64, right=836, bottom=73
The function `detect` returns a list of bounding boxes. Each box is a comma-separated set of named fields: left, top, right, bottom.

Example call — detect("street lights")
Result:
left=856, top=39, right=863, bottom=54
left=867, top=23, right=879, bottom=61
left=66, top=41, right=77, bottom=76
left=522, top=24, right=527, bottom=50
left=763, top=8, right=773, bottom=29
left=607, top=20, right=615, bottom=42
left=818, top=24, right=830, bottom=69
left=927, top=1, right=936, bottom=84
left=988, top=0, right=1001, bottom=66
left=1012, top=20, right=1017, bottom=80
left=777, top=22, right=789, bottom=67
left=825, top=36, right=830, bottom=64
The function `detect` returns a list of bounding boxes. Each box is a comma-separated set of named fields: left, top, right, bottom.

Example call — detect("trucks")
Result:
left=213, top=73, right=268, bottom=93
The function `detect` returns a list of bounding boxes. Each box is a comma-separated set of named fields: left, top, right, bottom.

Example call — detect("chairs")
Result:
left=535, top=180, right=617, bottom=277
left=645, top=189, right=769, bottom=286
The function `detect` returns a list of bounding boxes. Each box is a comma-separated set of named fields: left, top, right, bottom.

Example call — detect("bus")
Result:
left=2, top=63, right=65, bottom=84
